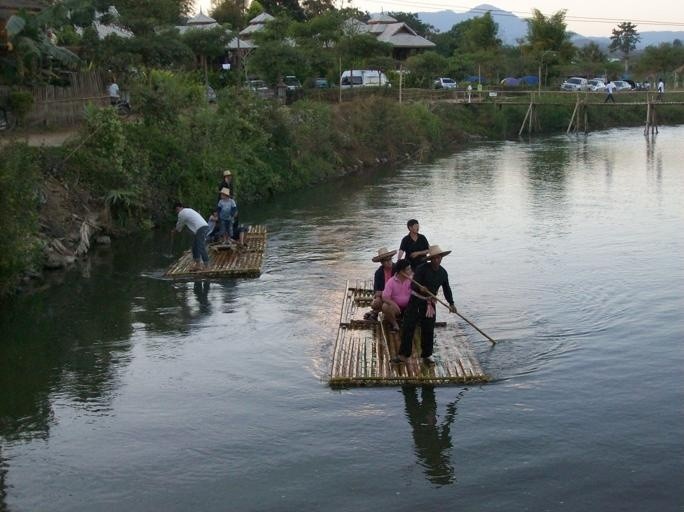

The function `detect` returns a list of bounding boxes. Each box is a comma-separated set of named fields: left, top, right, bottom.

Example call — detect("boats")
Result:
left=163, top=224, right=267, bottom=279
left=328, top=273, right=489, bottom=391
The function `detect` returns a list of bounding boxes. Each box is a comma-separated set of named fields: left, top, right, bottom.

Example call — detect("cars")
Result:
left=193, top=72, right=335, bottom=107
left=434, top=77, right=459, bottom=89
left=559, top=75, right=648, bottom=92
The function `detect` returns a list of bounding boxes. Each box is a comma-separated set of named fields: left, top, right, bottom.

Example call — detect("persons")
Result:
left=362, top=248, right=396, bottom=320
left=635, top=80, right=650, bottom=92
left=605, top=81, right=615, bottom=103
left=397, top=219, right=429, bottom=269
left=655, top=78, right=664, bottom=103
left=108, top=82, right=121, bottom=105
left=381, top=259, right=412, bottom=332
left=171, top=170, right=248, bottom=271
left=388, top=245, right=456, bottom=366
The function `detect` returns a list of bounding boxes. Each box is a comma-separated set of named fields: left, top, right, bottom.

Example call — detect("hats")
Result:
left=217, top=187, right=233, bottom=199
left=222, top=170, right=232, bottom=177
left=371, top=247, right=397, bottom=263
left=422, top=244, right=453, bottom=260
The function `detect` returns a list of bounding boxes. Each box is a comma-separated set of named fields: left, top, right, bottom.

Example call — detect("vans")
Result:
left=340, top=69, right=395, bottom=90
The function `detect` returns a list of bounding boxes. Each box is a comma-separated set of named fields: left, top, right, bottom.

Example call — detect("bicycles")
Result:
left=113, top=93, right=135, bottom=117
